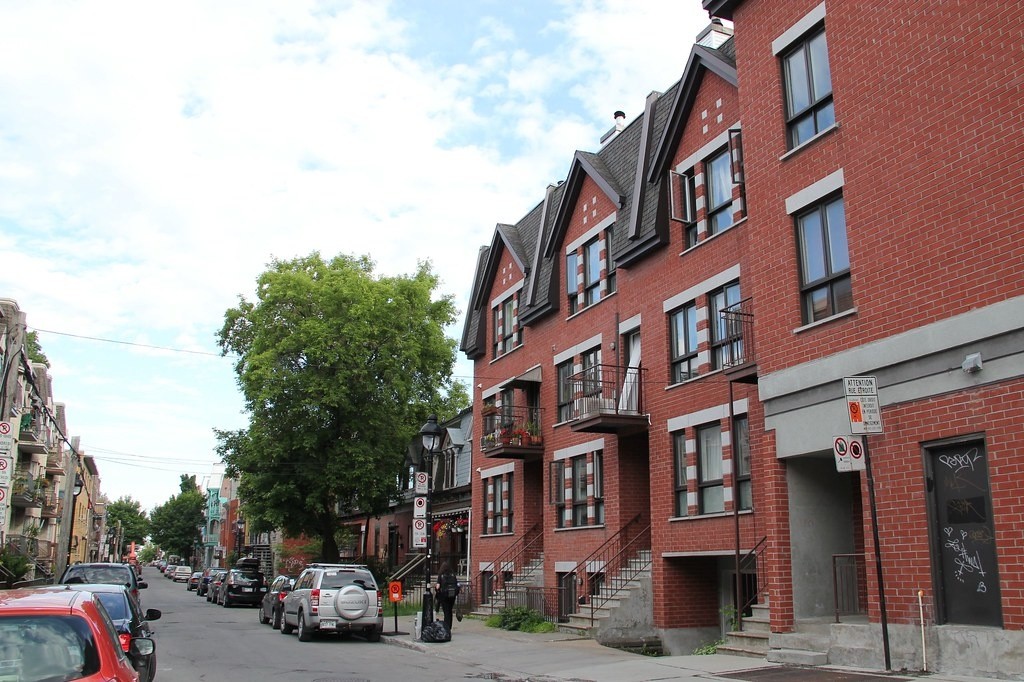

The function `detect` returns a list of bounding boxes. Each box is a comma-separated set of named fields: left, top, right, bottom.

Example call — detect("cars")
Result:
left=154, top=560, right=192, bottom=582
left=197, top=567, right=227, bottom=596
left=151, top=559, right=159, bottom=566
left=19, top=583, right=162, bottom=682
left=204, top=572, right=228, bottom=603
left=186, top=571, right=203, bottom=590
left=0, top=588, right=141, bottom=682
left=259, top=574, right=317, bottom=630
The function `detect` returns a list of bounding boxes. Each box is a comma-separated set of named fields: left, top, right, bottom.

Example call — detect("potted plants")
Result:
left=527, top=423, right=542, bottom=446
left=480, top=402, right=498, bottom=417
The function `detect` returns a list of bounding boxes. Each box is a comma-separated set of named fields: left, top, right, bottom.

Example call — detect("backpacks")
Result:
left=443, top=574, right=459, bottom=599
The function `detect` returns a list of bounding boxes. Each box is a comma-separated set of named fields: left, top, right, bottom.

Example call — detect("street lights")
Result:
left=237, top=515, right=245, bottom=560
left=418, top=414, right=445, bottom=635
left=108, top=528, right=114, bottom=562
left=193, top=535, right=198, bottom=571
left=66, top=473, right=83, bottom=569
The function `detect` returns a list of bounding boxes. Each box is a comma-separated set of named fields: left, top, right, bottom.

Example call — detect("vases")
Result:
left=511, top=437, right=519, bottom=447
left=486, top=440, right=495, bottom=447
left=500, top=437, right=511, bottom=446
left=456, top=526, right=464, bottom=532
left=451, top=528, right=457, bottom=533
left=522, top=437, right=530, bottom=448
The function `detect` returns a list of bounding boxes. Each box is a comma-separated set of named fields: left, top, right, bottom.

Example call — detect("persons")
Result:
left=353, top=547, right=358, bottom=561
left=436, top=562, right=460, bottom=632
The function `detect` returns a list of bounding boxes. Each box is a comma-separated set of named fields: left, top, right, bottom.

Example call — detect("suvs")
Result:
left=280, top=563, right=384, bottom=642
left=217, top=569, right=267, bottom=610
left=59, top=560, right=149, bottom=603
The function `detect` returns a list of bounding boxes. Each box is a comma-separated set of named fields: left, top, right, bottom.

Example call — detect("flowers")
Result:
left=521, top=428, right=530, bottom=437
left=434, top=519, right=457, bottom=541
left=499, top=429, right=509, bottom=438
left=457, top=519, right=468, bottom=527
left=511, top=429, right=519, bottom=438
left=486, top=434, right=495, bottom=441
